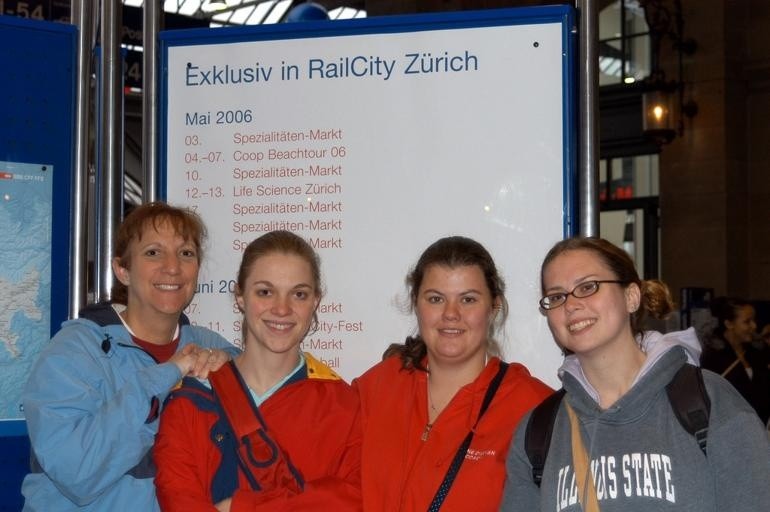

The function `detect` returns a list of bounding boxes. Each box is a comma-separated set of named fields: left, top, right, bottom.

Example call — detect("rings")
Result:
left=205, top=347, right=213, bottom=355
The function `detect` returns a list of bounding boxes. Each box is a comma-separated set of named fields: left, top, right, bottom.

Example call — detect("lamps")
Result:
left=642, top=79, right=698, bottom=144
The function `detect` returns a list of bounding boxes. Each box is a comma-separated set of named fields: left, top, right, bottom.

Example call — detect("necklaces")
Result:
left=429, top=359, right=439, bottom=417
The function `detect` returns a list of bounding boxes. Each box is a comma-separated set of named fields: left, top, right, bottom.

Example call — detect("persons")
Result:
left=498, top=237, right=766, bottom=510
left=153, top=230, right=361, bottom=511
left=351, top=237, right=557, bottom=511
left=703, top=298, right=766, bottom=424
left=23, top=202, right=244, bottom=512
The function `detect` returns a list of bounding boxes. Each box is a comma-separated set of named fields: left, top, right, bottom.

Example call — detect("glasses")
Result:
left=539, top=280, right=622, bottom=310
